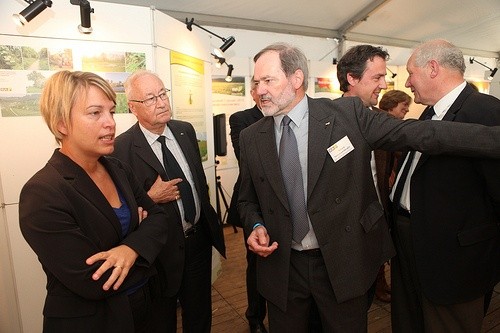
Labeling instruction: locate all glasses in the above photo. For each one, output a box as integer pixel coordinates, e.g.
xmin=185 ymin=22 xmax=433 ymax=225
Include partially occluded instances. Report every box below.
xmin=131 ymin=88 xmax=171 ymax=107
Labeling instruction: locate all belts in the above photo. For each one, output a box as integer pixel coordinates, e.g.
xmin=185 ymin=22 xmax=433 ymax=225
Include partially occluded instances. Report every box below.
xmin=184 ymin=221 xmax=203 ymax=239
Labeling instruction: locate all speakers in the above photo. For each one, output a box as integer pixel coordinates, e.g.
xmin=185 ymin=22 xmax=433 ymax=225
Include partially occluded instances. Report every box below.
xmin=213 ymin=114 xmax=228 ymax=156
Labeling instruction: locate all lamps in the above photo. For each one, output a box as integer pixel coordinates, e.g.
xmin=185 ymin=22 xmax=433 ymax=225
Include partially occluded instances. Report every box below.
xmin=70 ymin=0 xmax=94 ymax=34
xmin=12 ymin=0 xmax=52 ymax=25
xmin=470 ymin=57 xmax=498 ymax=80
xmin=211 ymin=53 xmax=234 ymax=82
xmin=185 ymin=17 xmax=236 ymax=53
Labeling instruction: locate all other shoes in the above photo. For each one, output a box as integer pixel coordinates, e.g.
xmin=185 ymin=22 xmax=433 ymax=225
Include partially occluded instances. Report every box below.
xmin=249 ymin=322 xmax=267 ymax=333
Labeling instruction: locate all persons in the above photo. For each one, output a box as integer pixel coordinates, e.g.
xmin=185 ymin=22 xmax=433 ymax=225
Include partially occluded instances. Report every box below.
xmin=105 ymin=71 xmax=226 ymax=333
xmin=389 ymin=38 xmax=500 ymax=333
xmin=337 ymin=45 xmax=388 ymax=309
xmin=228 ymin=75 xmax=267 ymax=333
xmin=19 ymin=71 xmax=171 ymax=333
xmin=238 ymin=42 xmax=500 ymax=333
xmin=375 ymin=90 xmax=412 ymax=303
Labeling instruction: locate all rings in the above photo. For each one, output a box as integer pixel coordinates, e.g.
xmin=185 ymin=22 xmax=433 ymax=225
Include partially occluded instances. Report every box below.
xmin=115 ymin=266 xmax=123 ymax=269
xmin=176 ymin=196 xmax=177 ymax=199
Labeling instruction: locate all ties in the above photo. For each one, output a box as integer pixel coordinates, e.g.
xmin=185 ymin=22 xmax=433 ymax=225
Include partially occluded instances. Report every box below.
xmin=157 ymin=135 xmax=196 ymax=225
xmin=391 ymin=106 xmax=435 ymax=231
xmin=279 ymin=116 xmax=310 ymax=246
